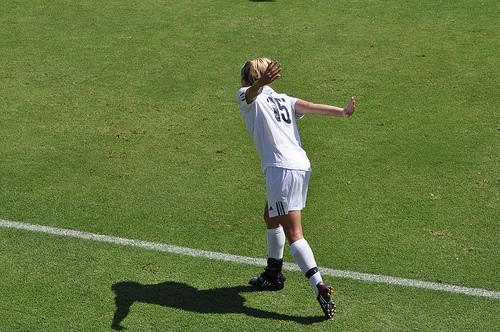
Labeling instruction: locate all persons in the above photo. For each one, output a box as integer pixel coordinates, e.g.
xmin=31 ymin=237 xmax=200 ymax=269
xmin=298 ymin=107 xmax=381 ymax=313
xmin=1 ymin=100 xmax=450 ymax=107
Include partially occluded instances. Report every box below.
xmin=236 ymin=58 xmax=357 ymax=319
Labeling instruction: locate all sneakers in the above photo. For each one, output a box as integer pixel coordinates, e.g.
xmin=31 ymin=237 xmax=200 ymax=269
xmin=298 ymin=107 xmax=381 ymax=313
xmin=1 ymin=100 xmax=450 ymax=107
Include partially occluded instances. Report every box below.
xmin=247 ymin=272 xmax=285 ymax=289
xmin=315 ymin=281 xmax=337 ymax=319
